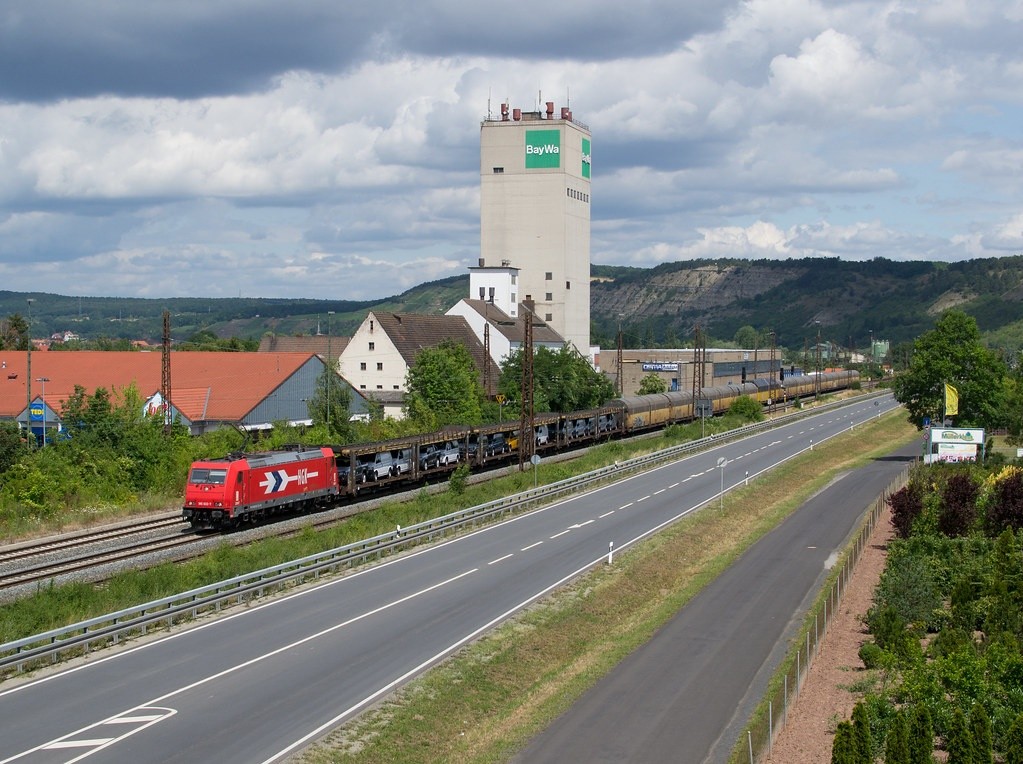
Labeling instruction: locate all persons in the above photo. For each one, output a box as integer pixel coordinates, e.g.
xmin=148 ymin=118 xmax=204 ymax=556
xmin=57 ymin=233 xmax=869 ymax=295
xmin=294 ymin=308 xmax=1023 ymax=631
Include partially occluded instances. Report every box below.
xmin=145 ymin=402 xmax=156 ymax=418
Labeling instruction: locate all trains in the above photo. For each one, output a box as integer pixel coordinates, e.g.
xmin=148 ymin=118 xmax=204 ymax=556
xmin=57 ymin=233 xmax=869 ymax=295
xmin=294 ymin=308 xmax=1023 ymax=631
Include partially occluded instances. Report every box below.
xmin=183 ymin=367 xmax=864 ymax=534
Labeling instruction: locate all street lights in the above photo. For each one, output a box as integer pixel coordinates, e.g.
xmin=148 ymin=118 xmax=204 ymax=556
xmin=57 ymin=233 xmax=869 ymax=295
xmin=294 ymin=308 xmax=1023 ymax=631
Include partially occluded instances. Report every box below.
xmin=618 ymin=314 xmax=625 ymax=350
xmin=28 ymin=299 xmax=42 ymax=448
xmin=815 ymin=320 xmax=821 ymax=397
xmin=869 ymin=330 xmax=873 ymax=390
xmin=327 ymin=310 xmax=336 ymax=438
xmin=35 ymin=378 xmax=50 ymax=449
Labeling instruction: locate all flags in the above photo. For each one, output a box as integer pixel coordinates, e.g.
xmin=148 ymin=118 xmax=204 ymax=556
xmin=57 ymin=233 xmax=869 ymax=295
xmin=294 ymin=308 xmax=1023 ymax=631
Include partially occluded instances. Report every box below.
xmin=945 ymin=384 xmax=959 ymax=416
